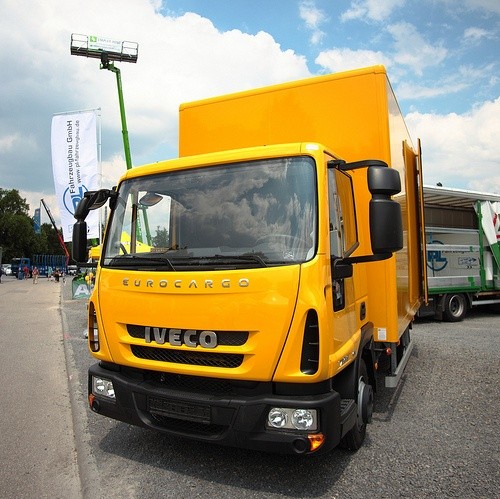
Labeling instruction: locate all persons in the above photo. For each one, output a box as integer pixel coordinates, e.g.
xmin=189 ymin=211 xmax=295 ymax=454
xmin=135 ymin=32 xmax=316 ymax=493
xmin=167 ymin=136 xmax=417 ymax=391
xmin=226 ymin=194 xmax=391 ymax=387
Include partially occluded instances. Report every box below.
xmin=47 ymin=264 xmax=65 ymax=285
xmin=0 ymin=268 xmax=2 ymax=282
xmin=23 ymin=266 xmax=29 ymax=280
xmin=32 ymin=267 xmax=39 ymax=284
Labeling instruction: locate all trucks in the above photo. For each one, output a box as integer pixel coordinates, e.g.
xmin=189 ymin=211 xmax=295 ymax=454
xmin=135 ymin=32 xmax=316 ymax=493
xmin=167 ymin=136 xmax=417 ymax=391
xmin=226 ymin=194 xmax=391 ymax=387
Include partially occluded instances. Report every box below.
xmin=0 ymin=255 xmax=67 ymax=278
xmin=68 ymin=63 xmax=500 ymax=457
